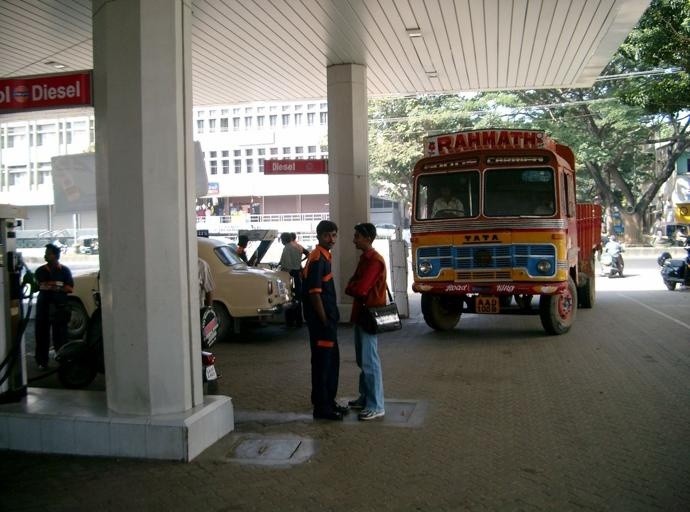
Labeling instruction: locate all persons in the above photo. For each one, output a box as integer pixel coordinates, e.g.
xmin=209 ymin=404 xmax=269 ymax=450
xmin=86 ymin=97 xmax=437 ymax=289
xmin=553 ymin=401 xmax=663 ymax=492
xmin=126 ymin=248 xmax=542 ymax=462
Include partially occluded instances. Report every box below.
xmin=34 ymin=243 xmax=75 ymax=370
xmin=604 ymin=234 xmax=625 ymax=276
xmin=235 ymin=237 xmax=248 ymax=262
xmin=431 ymin=186 xmax=465 ymax=218
xmin=302 ymin=220 xmax=349 ymax=421
xmin=198 ymin=256 xmax=215 ymax=309
xmin=344 ymin=223 xmax=387 ymax=421
xmin=280 ymin=233 xmax=301 ymax=282
xmin=291 ymin=232 xmax=310 ymax=261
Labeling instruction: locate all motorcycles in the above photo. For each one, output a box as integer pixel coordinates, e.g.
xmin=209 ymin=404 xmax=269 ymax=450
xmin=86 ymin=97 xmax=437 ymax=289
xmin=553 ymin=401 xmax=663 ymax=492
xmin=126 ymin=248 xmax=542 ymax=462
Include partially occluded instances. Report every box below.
xmin=53 ymin=290 xmax=219 ymax=398
xmin=601 ymin=242 xmax=626 ymax=277
xmin=657 ymin=247 xmax=690 ymax=291
xmin=668 ymin=235 xmax=690 ymax=246
xmin=58 ymin=237 xmax=98 ymax=255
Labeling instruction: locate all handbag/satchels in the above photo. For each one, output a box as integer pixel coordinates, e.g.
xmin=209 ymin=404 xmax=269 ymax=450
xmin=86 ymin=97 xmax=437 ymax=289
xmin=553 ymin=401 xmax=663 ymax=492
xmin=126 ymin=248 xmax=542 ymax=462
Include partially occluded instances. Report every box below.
xmin=363 ymin=302 xmax=403 ymax=335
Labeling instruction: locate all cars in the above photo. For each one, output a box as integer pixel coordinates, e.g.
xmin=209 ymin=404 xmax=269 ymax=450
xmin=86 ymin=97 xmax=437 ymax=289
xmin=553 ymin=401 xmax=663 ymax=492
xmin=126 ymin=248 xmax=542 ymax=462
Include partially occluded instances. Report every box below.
xmin=68 ymin=235 xmax=294 ymax=343
xmin=376 ymin=223 xmax=398 ymax=239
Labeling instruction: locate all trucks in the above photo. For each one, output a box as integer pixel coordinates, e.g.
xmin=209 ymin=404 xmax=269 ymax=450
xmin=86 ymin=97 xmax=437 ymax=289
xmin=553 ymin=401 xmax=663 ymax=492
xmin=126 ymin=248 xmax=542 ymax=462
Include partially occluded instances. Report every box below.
xmin=410 ymin=128 xmax=601 ymax=334
